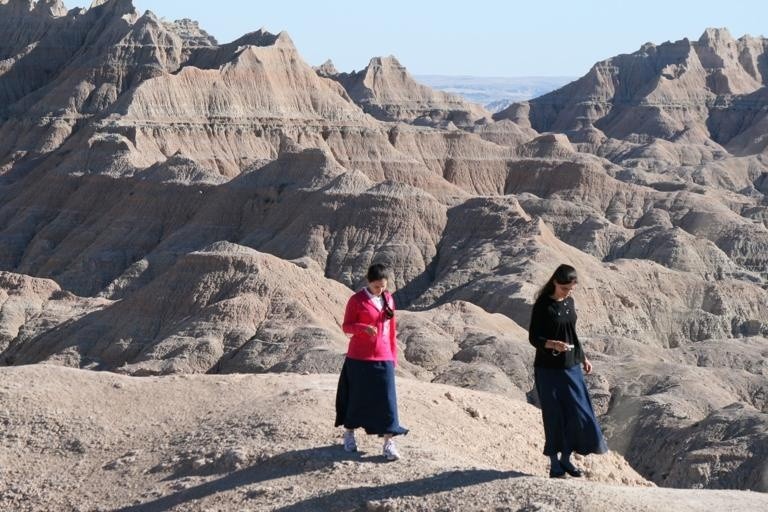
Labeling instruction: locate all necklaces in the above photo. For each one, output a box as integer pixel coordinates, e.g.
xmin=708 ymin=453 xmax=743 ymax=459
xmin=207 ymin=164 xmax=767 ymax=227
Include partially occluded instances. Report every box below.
xmin=553 ymin=298 xmax=563 ymax=316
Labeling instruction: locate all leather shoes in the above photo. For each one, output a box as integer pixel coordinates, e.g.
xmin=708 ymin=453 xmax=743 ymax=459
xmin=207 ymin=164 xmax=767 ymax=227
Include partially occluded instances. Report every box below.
xmin=558 ymin=461 xmax=584 ymax=477
xmin=550 ymin=468 xmax=566 ymax=478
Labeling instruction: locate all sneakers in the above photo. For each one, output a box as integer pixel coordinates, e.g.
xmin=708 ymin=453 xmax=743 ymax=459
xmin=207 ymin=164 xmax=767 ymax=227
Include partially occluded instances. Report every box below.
xmin=342 ymin=432 xmax=357 ymax=452
xmin=383 ymin=441 xmax=400 ymax=460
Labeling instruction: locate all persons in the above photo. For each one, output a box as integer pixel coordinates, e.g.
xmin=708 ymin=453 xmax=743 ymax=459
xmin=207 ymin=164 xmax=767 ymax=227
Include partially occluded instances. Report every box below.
xmin=335 ymin=263 xmax=408 ymax=461
xmin=528 ymin=265 xmax=606 ymax=477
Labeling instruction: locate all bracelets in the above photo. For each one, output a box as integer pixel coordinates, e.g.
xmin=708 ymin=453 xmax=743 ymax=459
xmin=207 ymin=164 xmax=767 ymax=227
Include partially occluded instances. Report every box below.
xmin=553 ymin=341 xmax=556 ymax=351
